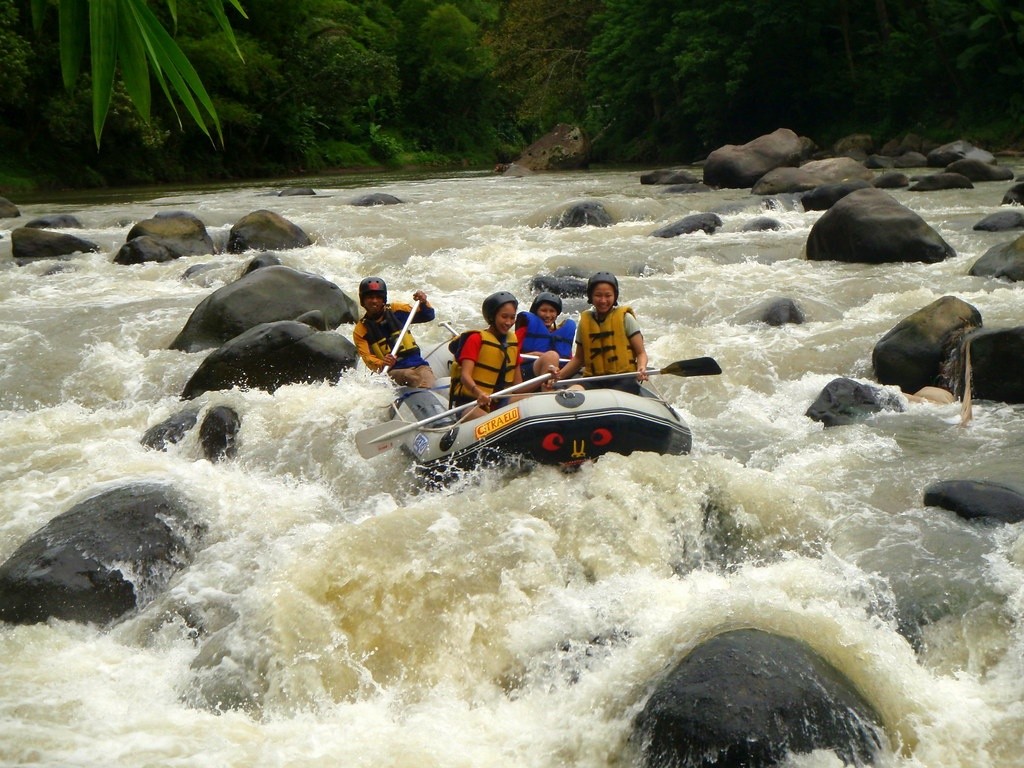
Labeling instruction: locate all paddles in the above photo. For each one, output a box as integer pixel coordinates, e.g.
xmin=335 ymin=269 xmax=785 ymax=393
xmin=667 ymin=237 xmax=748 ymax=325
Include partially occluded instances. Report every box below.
xmin=545 ymin=355 xmax=723 ymax=384
xmin=375 ymin=293 xmax=422 ymax=379
xmin=354 ymin=364 xmax=556 ymax=461
xmin=438 ymin=318 xmax=459 ymax=337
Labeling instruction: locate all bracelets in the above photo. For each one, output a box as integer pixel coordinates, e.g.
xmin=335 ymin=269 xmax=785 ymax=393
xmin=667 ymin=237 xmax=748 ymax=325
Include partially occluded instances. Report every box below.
xmin=470 ymin=384 xmax=479 ymax=394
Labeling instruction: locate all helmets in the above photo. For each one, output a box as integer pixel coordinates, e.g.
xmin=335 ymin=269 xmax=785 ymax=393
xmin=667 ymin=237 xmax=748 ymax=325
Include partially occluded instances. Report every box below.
xmin=587 ymin=271 xmax=619 ymax=306
xmin=482 ymin=292 xmax=518 ymax=326
xmin=360 ymin=277 xmax=387 ymax=306
xmin=530 ymin=292 xmax=562 ymax=316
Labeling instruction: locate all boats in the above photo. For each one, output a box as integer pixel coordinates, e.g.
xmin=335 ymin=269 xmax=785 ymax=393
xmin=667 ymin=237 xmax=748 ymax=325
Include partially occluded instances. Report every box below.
xmin=385 ymin=375 xmax=692 ymax=489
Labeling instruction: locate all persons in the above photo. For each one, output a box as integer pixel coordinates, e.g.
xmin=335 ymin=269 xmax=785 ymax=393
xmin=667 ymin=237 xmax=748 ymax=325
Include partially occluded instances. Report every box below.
xmin=443 ymin=292 xmax=562 ymax=424
xmin=349 ymin=275 xmax=450 ymax=426
xmin=508 ymin=289 xmax=576 ymax=393
xmin=554 ymin=271 xmax=649 ymax=394
